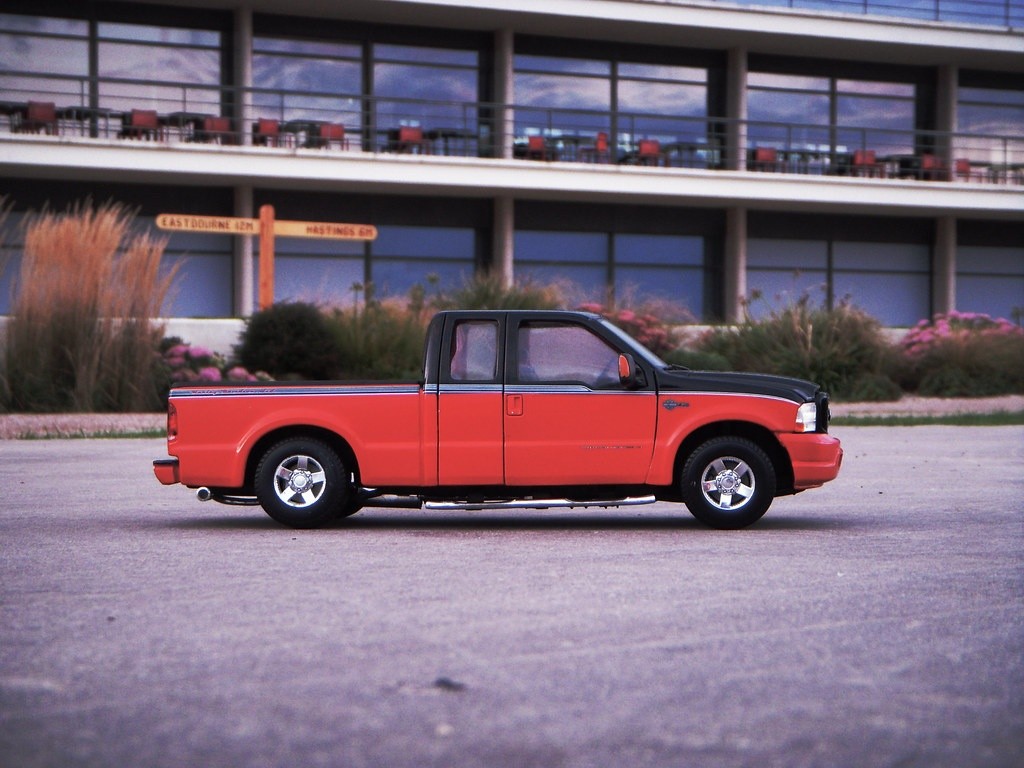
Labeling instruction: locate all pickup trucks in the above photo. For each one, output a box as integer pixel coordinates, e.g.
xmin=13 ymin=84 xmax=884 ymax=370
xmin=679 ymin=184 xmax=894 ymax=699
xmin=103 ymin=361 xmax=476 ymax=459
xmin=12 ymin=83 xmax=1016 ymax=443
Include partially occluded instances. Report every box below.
xmin=152 ymin=308 xmax=843 ymax=529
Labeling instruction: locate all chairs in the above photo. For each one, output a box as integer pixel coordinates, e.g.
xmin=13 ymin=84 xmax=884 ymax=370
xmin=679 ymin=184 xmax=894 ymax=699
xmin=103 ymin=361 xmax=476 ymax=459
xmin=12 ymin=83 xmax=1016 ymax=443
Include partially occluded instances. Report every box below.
xmin=519 ymin=349 xmax=539 ymax=382
xmin=0 ymin=100 xmax=1023 ymax=187
